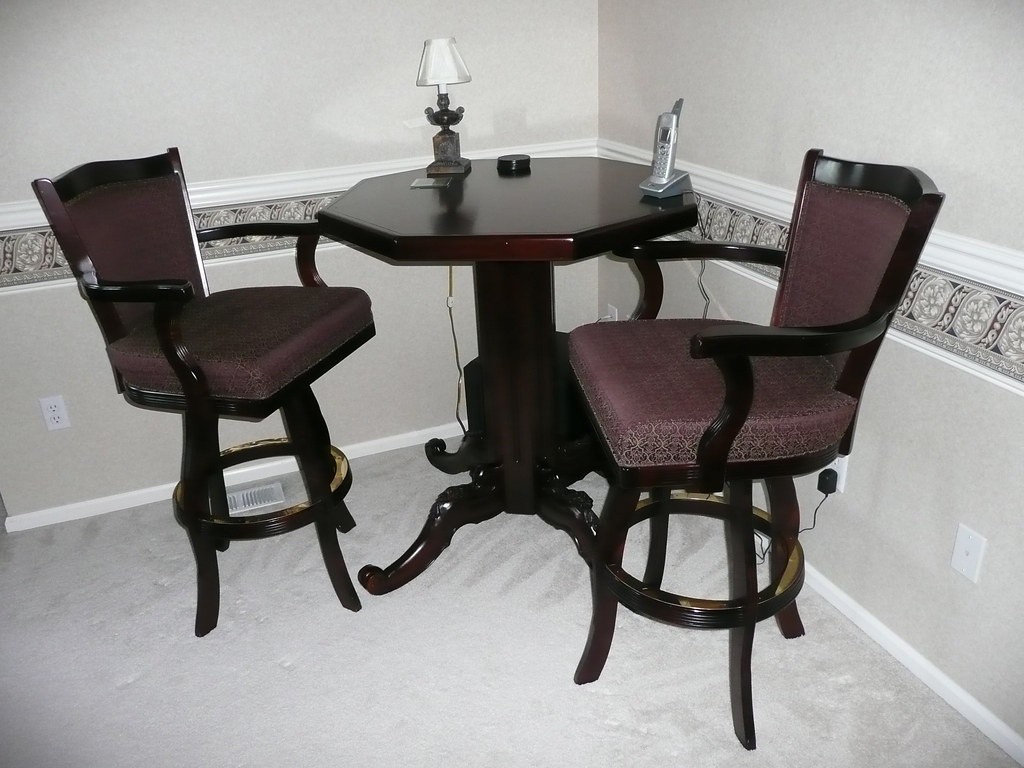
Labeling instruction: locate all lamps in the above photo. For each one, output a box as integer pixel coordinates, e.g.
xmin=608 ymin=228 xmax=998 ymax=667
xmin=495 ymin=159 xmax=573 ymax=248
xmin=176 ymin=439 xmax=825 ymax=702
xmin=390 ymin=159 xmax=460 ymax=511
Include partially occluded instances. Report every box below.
xmin=415 ymin=36 xmax=472 ymax=177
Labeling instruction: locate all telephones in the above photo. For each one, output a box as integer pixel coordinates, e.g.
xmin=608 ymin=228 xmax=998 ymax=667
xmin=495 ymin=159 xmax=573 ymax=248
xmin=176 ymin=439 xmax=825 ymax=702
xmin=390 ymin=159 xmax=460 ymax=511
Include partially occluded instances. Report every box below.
xmin=637 ymin=97 xmax=692 ymax=199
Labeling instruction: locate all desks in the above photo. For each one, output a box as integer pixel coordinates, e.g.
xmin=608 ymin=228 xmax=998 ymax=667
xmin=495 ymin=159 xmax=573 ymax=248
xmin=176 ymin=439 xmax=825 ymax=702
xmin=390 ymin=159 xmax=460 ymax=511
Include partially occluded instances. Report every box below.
xmin=315 ymin=157 xmax=699 ymax=596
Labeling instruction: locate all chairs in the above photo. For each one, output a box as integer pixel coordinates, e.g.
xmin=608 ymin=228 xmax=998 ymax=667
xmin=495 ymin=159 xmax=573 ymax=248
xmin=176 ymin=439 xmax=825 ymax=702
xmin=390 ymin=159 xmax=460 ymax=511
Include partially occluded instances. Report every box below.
xmin=573 ymin=148 xmax=945 ymax=752
xmin=30 ymin=145 xmax=377 ymax=638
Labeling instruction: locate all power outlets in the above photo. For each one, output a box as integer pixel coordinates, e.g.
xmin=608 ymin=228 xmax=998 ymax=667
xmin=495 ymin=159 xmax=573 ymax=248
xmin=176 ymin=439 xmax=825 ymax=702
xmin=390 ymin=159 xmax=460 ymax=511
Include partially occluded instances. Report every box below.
xmin=950 ymin=522 xmax=986 ymax=582
xmin=819 ymin=454 xmax=848 ymax=494
xmin=604 ymin=304 xmax=617 ymax=322
xmin=39 ymin=394 xmax=69 ymax=431
